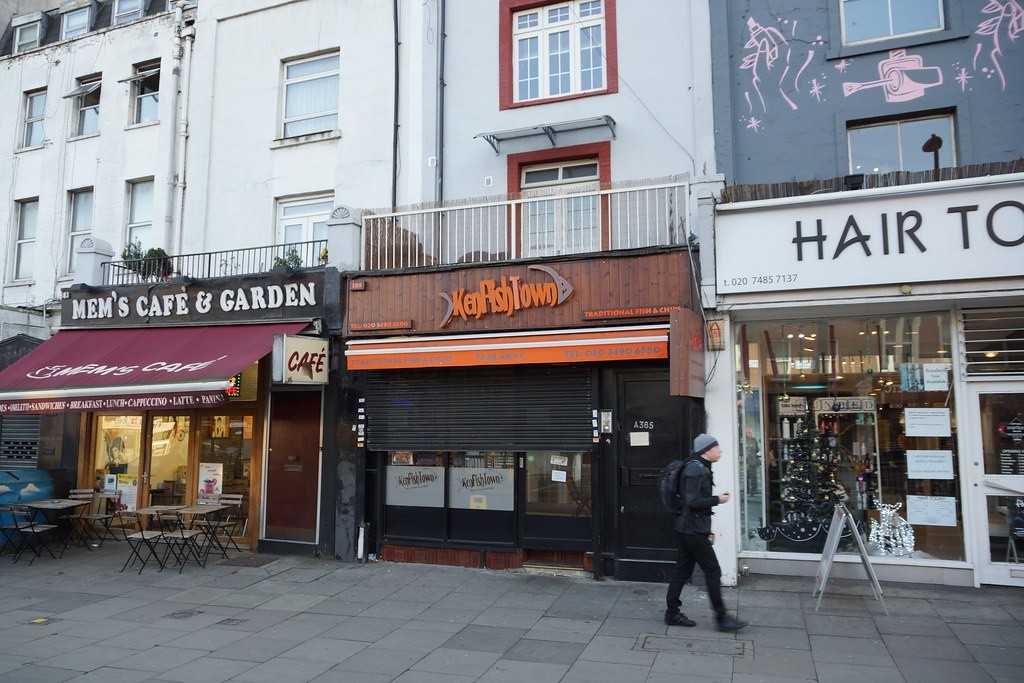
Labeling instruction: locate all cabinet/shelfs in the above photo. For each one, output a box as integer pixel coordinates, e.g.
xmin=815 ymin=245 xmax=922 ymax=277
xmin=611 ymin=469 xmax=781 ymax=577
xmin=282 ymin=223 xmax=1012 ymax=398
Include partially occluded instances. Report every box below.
xmin=774 ymin=396 xmax=884 ymax=543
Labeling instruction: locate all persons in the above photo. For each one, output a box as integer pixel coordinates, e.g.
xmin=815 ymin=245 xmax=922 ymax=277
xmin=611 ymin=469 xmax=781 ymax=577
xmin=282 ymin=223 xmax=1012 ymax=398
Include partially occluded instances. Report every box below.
xmin=166 ymin=416 xmax=178 ymax=439
xmin=657 ymin=433 xmax=750 ymax=632
xmin=394 ymin=451 xmax=411 ymax=463
xmin=212 ymin=416 xmax=225 ymax=437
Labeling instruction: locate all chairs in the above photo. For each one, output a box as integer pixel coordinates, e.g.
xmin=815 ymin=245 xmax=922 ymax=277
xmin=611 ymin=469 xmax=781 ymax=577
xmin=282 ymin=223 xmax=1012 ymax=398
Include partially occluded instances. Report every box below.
xmin=0 ymin=503 xmax=60 ymax=564
xmin=120 ymin=491 xmax=246 ymax=577
xmin=63 ymin=487 xmax=125 ymax=552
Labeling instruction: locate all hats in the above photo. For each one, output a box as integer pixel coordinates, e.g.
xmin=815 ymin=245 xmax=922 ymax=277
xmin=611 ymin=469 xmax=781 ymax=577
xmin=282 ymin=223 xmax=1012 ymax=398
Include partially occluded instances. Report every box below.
xmin=693 ymin=433 xmax=718 ymax=455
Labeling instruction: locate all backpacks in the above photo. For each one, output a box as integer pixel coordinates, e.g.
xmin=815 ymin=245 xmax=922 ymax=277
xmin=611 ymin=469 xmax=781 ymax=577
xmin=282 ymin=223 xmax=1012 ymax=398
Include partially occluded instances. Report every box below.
xmin=659 ymin=460 xmax=708 ymax=508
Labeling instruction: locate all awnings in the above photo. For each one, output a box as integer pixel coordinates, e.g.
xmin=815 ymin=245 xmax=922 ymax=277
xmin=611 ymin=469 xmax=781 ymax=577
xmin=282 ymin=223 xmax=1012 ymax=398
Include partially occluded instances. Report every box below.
xmin=0 ymin=320 xmax=313 ymax=415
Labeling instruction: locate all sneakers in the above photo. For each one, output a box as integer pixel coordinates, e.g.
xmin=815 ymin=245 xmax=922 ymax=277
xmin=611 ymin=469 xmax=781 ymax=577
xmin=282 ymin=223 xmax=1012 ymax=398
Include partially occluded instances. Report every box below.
xmin=665 ymin=612 xmax=696 ymax=627
xmin=718 ymin=616 xmax=747 ymax=631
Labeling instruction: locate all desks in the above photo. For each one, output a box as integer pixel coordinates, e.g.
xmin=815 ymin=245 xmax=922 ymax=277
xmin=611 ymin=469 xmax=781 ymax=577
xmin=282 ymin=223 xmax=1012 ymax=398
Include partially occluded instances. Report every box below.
xmin=134 ymin=505 xmax=233 ymax=561
xmin=10 ymin=498 xmax=91 ymax=558
